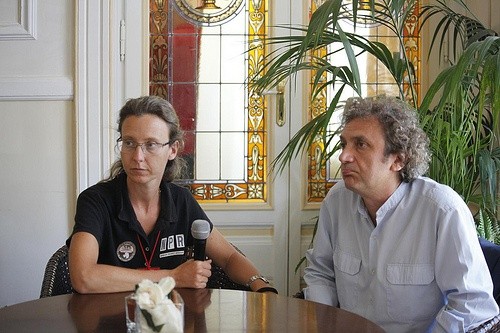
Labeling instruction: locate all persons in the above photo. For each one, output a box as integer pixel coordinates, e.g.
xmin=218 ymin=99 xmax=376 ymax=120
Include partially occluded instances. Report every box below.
xmin=68 ymin=288 xmax=212 ymax=333
xmin=302 ymin=95 xmax=500 ymax=333
xmin=65 ymin=96 xmax=279 ymax=295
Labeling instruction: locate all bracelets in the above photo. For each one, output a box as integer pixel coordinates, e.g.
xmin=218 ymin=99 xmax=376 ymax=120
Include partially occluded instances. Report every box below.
xmin=257 ymin=287 xmax=278 ymax=294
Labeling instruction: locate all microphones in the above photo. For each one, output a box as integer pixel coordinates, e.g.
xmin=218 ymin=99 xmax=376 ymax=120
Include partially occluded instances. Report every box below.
xmin=190 ymin=219 xmax=210 ymax=260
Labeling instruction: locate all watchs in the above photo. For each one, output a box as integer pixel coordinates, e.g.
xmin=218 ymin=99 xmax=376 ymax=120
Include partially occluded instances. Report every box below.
xmin=245 ymin=274 xmax=269 ymax=290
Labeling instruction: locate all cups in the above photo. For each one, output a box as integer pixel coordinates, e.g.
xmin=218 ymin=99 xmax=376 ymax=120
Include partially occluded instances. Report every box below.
xmin=123 ymin=289 xmax=185 ymax=333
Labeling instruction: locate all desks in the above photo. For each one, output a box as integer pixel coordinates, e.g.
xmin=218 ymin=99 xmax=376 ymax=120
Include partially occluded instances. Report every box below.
xmin=0 ymin=287 xmax=386 ymax=333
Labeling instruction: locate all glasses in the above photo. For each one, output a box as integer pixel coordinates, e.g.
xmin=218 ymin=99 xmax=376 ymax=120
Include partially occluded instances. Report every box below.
xmin=116 ymin=136 xmax=172 ymax=157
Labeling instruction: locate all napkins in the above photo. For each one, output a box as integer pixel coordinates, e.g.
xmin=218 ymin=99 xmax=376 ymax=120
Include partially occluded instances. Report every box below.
xmin=134 ymin=276 xmax=182 ymax=333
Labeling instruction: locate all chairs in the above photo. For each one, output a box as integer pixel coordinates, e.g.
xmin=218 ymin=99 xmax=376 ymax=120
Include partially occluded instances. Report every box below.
xmin=39 ymin=245 xmax=251 ymax=298
